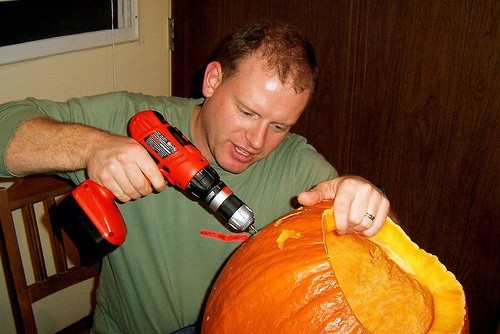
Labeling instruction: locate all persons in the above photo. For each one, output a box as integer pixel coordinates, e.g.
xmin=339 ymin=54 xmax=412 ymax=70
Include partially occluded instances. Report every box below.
xmin=0 ymin=23 xmax=392 ymax=333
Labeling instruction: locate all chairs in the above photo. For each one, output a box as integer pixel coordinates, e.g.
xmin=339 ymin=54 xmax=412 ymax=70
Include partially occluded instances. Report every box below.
xmin=0 ymin=172 xmax=103 ymax=334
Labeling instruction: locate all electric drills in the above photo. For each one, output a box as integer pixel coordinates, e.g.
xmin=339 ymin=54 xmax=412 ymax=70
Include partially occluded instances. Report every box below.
xmin=47 ymin=109 xmax=258 ymax=272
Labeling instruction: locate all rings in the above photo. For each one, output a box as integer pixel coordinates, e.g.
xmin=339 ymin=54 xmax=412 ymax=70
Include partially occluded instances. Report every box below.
xmin=364 ymin=212 xmax=376 ymax=221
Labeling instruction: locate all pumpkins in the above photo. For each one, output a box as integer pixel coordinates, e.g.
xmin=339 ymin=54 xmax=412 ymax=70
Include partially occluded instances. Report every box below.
xmin=200 ymin=199 xmax=471 ymax=334
